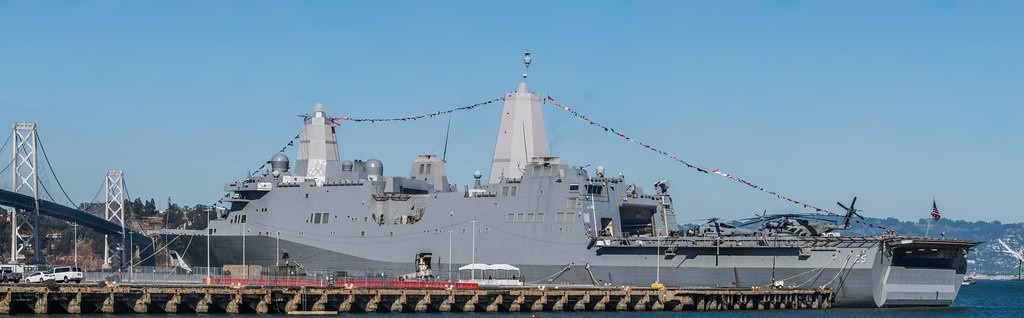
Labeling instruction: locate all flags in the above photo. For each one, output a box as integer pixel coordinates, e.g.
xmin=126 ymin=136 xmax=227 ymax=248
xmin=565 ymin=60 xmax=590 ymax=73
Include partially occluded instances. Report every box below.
xmin=931 ymin=200 xmax=941 ymax=222
xmin=331 ymin=120 xmax=341 ymax=128
xmin=548 ymin=95 xmax=554 ymax=103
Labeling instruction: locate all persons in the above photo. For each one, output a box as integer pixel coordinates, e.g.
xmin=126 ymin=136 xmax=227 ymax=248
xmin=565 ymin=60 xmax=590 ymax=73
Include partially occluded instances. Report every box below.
xmin=686 ymin=229 xmax=698 ymax=237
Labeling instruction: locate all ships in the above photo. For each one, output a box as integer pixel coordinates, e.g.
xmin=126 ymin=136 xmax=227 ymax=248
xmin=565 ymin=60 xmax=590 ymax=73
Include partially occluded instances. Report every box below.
xmin=147 ymin=52 xmax=989 ymax=309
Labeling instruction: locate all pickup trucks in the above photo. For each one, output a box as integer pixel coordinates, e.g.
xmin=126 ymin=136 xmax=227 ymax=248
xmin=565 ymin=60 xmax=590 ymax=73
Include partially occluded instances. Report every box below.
xmin=0 ymin=269 xmax=22 ymax=283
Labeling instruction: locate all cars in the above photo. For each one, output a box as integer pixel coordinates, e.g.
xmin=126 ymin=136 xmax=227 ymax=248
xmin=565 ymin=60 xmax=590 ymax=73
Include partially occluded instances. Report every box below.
xmin=24 ymin=271 xmax=55 ymax=284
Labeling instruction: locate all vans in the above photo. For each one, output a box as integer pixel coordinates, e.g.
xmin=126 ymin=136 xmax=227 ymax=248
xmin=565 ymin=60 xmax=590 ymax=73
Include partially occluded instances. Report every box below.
xmin=49 ymin=265 xmax=83 ymax=284
